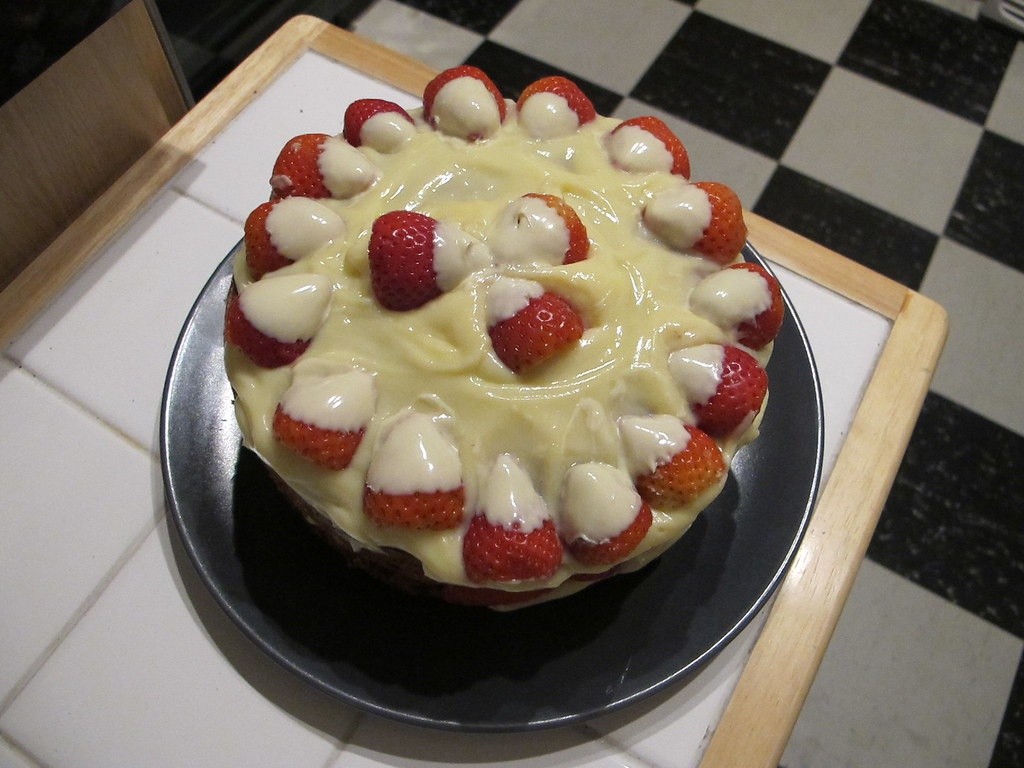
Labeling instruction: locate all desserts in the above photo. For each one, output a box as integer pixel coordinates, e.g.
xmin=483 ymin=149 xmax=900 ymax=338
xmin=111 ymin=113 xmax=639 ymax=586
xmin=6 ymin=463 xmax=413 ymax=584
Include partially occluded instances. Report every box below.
xmin=224 ymin=63 xmax=782 ymax=613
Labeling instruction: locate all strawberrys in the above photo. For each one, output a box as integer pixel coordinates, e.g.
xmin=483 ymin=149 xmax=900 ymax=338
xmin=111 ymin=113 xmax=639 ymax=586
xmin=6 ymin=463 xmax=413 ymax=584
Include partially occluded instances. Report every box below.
xmin=225 ymin=68 xmax=784 ymax=581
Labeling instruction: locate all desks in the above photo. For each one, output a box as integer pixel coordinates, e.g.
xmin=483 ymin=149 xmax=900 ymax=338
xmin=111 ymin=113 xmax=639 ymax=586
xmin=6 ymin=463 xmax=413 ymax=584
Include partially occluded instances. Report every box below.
xmin=229 ymin=0 xmax=1024 ymax=767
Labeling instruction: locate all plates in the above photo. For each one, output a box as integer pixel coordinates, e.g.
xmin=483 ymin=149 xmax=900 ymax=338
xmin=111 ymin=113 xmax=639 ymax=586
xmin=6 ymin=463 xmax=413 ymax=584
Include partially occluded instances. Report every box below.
xmin=164 ymin=230 xmax=824 ymax=735
